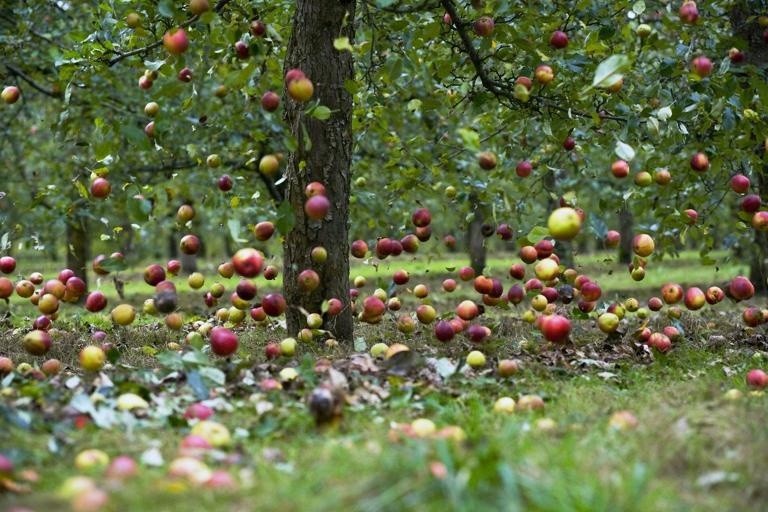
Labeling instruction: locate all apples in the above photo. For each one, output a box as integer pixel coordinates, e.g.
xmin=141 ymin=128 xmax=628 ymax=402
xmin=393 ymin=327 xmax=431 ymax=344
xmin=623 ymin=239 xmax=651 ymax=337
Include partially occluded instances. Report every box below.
xmin=0 ymin=1 xmax=768 ymax=512
